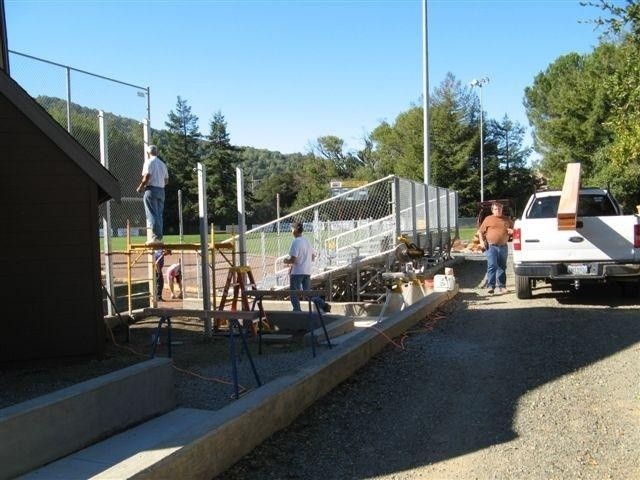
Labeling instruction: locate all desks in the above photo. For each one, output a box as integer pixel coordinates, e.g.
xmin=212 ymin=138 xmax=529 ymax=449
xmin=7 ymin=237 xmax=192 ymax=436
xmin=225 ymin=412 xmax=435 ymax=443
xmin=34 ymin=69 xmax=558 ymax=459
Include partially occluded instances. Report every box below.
xmin=471 ymin=76 xmax=491 ymax=200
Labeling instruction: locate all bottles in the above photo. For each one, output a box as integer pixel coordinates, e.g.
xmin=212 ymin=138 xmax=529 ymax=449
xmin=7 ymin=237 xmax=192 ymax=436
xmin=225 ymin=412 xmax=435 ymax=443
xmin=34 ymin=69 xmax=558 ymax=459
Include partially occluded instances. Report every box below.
xmin=289 ymin=223 xmax=303 ymax=231
xmin=166 ymin=249 xmax=172 ymax=255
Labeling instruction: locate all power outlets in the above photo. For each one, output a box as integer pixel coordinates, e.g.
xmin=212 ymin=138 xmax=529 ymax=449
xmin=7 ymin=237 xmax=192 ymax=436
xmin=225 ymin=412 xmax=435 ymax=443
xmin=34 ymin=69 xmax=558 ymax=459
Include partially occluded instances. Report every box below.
xmin=513 ymin=188 xmax=640 ymax=300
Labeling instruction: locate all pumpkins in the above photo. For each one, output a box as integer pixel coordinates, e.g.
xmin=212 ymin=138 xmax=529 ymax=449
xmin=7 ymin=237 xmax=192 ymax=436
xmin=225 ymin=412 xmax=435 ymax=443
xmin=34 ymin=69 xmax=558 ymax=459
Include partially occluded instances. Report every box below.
xmin=214 ymin=267 xmax=273 ymax=342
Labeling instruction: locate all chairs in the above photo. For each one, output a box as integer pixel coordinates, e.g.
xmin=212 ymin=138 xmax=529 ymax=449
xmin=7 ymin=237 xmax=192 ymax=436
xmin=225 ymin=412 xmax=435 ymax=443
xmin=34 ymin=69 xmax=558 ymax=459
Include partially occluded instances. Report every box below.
xmin=156 ymin=293 xmax=184 ymax=302
xmin=322 ymin=303 xmax=331 ymax=312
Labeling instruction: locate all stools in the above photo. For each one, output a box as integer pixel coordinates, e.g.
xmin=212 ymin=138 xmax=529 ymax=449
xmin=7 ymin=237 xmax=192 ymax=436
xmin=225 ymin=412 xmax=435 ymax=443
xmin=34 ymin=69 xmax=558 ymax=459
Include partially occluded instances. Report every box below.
xmin=487 ymin=243 xmax=507 ymax=247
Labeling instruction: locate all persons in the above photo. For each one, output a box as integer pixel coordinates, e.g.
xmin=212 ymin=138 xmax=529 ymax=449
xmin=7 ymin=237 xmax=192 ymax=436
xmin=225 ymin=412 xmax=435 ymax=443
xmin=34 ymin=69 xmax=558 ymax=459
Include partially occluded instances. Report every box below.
xmin=154 ymin=249 xmax=173 ymax=302
xmin=136 ymin=144 xmax=169 ymax=246
xmin=283 ymin=222 xmax=331 ymax=315
xmin=478 ymin=201 xmax=514 ymax=294
xmin=167 ymin=258 xmax=183 ymax=298
